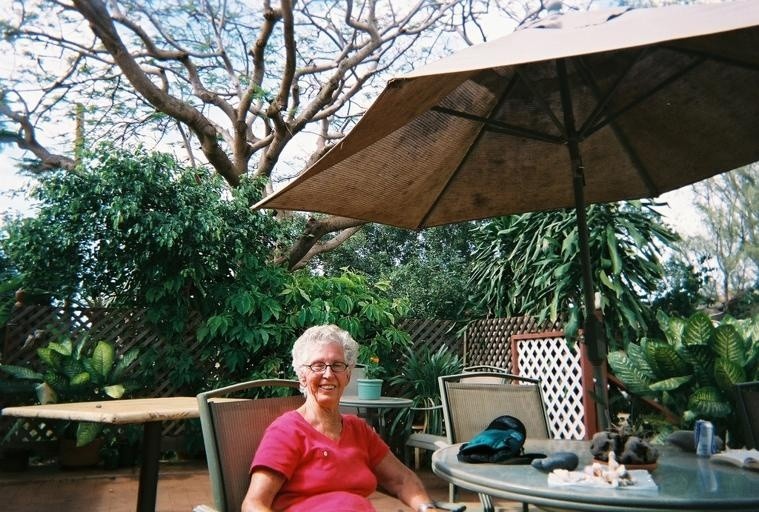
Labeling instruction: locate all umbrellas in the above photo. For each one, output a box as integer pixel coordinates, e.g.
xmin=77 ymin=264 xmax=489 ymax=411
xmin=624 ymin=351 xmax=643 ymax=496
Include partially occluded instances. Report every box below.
xmin=248 ymin=0 xmax=759 ymax=431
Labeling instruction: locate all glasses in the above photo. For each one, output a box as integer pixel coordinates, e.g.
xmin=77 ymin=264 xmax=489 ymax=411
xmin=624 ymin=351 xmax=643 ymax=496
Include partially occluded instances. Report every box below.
xmin=302 ymin=360 xmax=349 ymax=372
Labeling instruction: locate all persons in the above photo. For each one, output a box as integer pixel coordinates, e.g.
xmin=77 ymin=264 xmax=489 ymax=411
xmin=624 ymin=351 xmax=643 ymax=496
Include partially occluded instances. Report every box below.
xmin=242 ymin=325 xmax=447 ymax=512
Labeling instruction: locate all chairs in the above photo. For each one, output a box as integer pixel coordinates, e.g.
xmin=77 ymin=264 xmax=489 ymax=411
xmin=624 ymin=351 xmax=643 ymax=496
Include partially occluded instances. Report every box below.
xmin=405 ymin=366 xmax=554 ymax=511
xmin=197 ymin=377 xmax=466 ymax=511
xmin=334 ymin=366 xmax=368 ymax=419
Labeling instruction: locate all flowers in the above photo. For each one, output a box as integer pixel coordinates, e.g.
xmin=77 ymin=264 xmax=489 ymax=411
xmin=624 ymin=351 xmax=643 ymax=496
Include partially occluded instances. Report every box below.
xmin=365 ymin=356 xmax=386 ymax=379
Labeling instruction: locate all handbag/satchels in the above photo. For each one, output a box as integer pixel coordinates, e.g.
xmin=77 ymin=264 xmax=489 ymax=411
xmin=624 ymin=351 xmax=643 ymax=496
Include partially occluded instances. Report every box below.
xmin=457 ymin=415 xmax=546 ymax=465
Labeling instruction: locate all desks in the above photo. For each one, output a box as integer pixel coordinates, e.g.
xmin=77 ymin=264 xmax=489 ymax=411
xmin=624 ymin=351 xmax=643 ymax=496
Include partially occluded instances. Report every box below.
xmin=337 ymin=393 xmax=414 ymax=437
xmin=2 ymin=395 xmax=254 ymax=512
xmin=429 ymin=439 xmax=759 ymax=510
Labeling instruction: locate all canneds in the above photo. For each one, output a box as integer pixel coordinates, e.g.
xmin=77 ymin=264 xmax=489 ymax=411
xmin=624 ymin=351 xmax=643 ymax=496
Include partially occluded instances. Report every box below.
xmin=694 ymin=420 xmax=716 ymax=457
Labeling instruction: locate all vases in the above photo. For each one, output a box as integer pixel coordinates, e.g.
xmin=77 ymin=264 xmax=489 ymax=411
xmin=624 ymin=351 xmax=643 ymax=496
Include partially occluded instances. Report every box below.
xmin=355 ymin=377 xmax=385 ymax=400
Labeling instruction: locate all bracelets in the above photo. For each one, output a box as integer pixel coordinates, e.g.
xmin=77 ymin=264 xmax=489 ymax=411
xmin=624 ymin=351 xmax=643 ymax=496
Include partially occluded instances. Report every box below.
xmin=418 ymin=503 xmax=436 ymax=512
